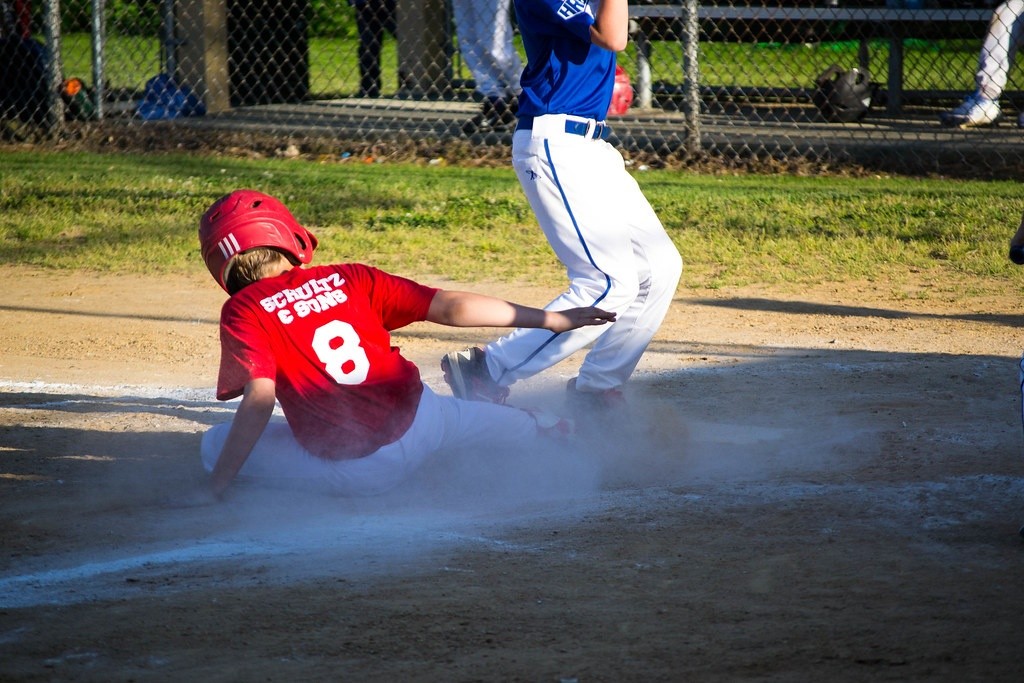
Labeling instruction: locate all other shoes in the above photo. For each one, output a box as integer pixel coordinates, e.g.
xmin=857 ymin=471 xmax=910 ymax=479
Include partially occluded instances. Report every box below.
xmin=472 ymin=98 xmax=519 ymax=130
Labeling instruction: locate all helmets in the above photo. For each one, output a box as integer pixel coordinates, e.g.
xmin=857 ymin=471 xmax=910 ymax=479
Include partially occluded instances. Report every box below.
xmin=198 ymin=188 xmax=318 ymax=296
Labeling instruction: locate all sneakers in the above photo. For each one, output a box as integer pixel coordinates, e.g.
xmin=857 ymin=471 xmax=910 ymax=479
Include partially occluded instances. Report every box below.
xmin=566 ymin=378 xmax=629 ymax=426
xmin=939 ymin=96 xmax=1003 ymax=127
xmin=440 ymin=345 xmax=510 ymax=405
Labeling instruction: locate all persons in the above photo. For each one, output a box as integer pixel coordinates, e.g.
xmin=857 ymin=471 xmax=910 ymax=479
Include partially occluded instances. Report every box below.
xmin=171 ymin=189 xmax=633 ymax=510
xmin=345 ymin=0 xmax=396 ymax=98
xmin=440 ymin=0 xmax=684 ymax=416
xmin=942 ymin=0 xmax=1024 ymax=267
xmin=452 ymin=0 xmax=524 ymax=135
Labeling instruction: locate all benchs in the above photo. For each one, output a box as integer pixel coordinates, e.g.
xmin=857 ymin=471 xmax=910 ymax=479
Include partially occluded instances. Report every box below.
xmin=628 ymin=4 xmax=995 ymax=115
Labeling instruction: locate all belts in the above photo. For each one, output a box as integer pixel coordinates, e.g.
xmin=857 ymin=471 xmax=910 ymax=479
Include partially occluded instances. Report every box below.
xmin=516 ymin=115 xmax=611 ymax=140
